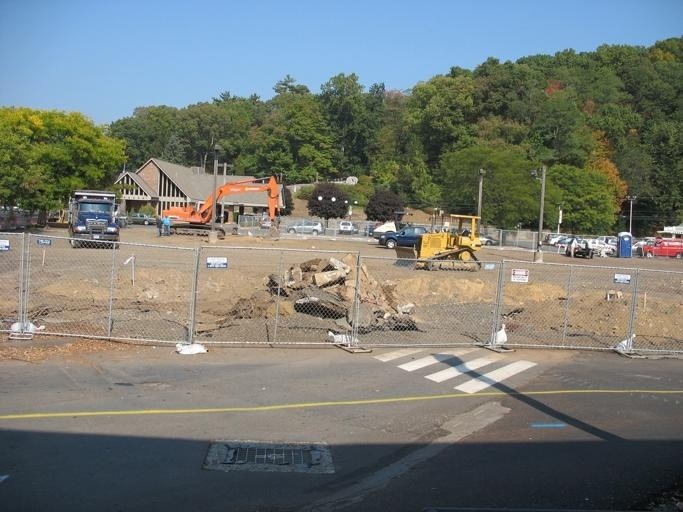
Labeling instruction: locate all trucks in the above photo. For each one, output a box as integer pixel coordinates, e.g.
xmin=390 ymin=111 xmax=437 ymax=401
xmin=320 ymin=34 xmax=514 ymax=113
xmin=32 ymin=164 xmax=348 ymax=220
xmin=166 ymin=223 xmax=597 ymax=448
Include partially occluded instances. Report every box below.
xmin=66 ymin=187 xmax=119 ymax=249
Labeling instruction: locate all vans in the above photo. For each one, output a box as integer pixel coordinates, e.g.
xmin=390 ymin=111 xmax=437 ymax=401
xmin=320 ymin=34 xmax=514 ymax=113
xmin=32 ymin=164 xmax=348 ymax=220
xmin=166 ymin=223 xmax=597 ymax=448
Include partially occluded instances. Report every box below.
xmin=639 ymin=238 xmax=682 ymax=260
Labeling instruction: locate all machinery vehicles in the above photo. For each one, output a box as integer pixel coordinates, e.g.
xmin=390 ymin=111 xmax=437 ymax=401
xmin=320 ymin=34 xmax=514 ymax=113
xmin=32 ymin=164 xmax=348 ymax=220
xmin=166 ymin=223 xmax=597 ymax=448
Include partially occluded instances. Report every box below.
xmin=393 ymin=211 xmax=483 ymax=273
xmin=162 ymin=173 xmax=283 ymax=236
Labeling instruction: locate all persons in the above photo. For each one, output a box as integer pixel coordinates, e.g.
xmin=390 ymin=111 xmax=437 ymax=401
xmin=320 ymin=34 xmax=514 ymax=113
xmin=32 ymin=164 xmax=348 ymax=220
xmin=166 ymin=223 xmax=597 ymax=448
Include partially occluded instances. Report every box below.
xmin=570 ymin=236 xmax=579 ymax=257
xmin=156 ymin=215 xmax=163 ymax=237
xmin=162 ymin=214 xmax=171 ymax=236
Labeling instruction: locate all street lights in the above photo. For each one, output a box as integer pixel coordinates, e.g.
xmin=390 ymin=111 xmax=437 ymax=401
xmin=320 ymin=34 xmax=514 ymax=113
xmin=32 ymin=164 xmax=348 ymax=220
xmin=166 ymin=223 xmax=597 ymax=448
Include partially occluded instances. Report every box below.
xmin=477 ymin=167 xmax=486 ymax=232
xmin=531 ymin=165 xmax=547 ymax=263
xmin=626 ymin=194 xmax=638 ymax=234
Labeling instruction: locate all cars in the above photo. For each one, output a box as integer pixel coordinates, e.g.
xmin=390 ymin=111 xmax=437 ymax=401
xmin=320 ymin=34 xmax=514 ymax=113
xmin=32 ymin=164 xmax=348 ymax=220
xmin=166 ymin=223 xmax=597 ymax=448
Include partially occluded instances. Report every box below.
xmin=546 ymin=231 xmax=621 ymax=258
xmin=259 ymin=211 xmax=360 ymax=238
xmin=378 ymin=226 xmax=428 ymax=249
xmin=479 ymin=233 xmax=499 ymax=246
xmin=132 ymin=211 xmax=156 ymax=226
